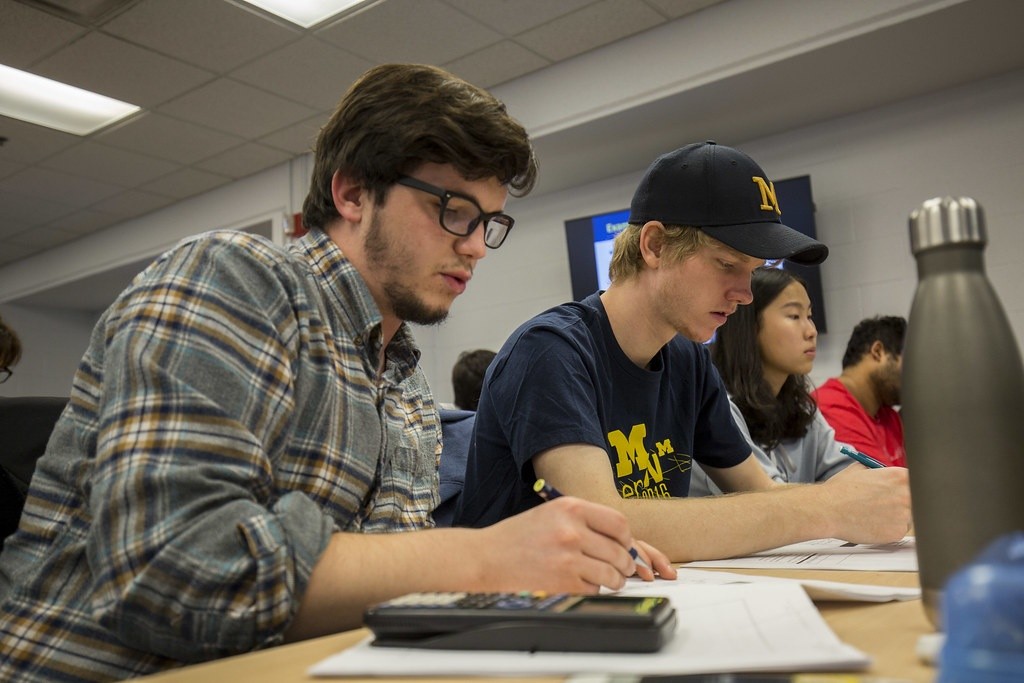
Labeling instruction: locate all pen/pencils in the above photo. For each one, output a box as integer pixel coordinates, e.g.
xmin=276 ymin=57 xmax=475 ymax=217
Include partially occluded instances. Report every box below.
xmin=531 ymin=478 xmax=655 ymax=574
xmin=840 ymin=446 xmax=888 ymax=468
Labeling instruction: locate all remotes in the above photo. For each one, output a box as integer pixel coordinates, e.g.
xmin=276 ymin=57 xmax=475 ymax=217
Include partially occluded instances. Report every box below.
xmin=365 ymin=588 xmax=677 ymax=654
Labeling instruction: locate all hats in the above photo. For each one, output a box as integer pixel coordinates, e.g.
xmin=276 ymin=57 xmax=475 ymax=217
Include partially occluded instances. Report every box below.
xmin=627 ymin=140 xmax=829 ymax=266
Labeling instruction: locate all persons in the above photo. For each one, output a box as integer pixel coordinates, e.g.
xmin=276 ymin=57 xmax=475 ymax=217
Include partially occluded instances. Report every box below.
xmin=0 ymin=65 xmax=678 ymax=682
xmin=452 ymin=140 xmax=916 ymax=563
xmin=687 ymin=265 xmax=860 ymax=496
xmin=432 ymin=350 xmax=498 ymax=528
xmin=811 ymin=315 xmax=909 ymax=467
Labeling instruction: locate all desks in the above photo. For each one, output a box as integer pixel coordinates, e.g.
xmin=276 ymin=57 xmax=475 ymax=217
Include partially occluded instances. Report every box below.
xmin=122 ymin=535 xmax=943 ymax=683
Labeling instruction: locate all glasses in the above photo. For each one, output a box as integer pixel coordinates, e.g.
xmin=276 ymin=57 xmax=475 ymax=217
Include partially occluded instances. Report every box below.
xmin=0 ymin=365 xmax=13 ymax=384
xmin=393 ymin=174 xmax=514 ymax=250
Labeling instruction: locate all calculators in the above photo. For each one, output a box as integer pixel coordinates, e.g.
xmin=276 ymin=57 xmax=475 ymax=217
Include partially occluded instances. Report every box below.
xmin=362 ymin=588 xmax=679 ymax=653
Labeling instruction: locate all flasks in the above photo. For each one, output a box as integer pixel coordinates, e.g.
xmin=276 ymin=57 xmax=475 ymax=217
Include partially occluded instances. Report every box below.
xmin=903 ymin=196 xmax=1023 ymax=626
